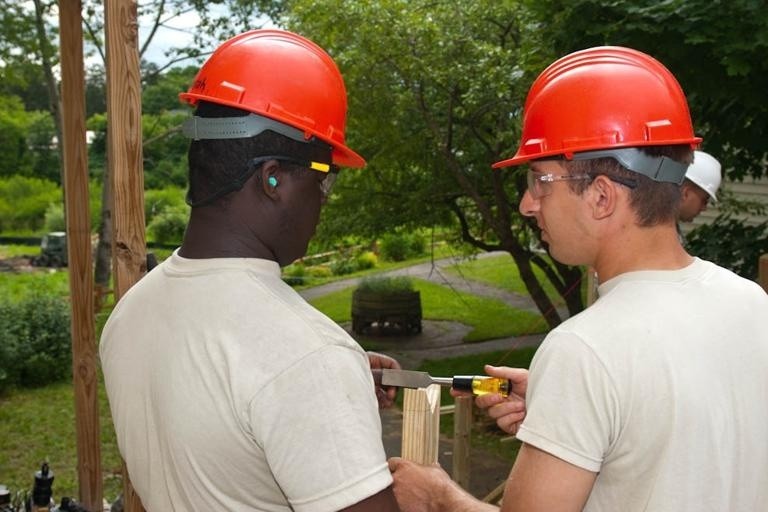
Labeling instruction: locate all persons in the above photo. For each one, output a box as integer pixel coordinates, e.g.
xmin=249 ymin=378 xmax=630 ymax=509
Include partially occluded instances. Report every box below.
xmin=384 ymin=43 xmax=767 ymax=512
xmin=678 ymin=151 xmax=722 ymax=224
xmin=94 ymin=29 xmax=399 ymax=512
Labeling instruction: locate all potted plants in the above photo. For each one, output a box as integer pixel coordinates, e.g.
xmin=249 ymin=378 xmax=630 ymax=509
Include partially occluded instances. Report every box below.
xmin=348 ymin=271 xmax=422 ymax=336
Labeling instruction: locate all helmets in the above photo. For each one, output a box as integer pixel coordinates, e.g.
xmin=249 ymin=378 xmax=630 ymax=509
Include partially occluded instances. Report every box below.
xmin=685 ymin=152 xmax=722 ymax=205
xmin=492 ymin=47 xmax=701 ymax=168
xmin=178 ymin=28 xmax=367 ymax=168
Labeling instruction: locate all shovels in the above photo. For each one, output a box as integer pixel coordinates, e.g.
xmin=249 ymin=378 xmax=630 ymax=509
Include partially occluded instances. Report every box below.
xmin=382 ymin=368 xmax=512 ymax=397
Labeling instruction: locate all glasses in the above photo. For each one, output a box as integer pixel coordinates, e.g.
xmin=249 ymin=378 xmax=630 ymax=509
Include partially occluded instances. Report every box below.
xmin=247 ymin=154 xmax=340 ymax=195
xmin=526 ymin=169 xmax=636 ymax=200
